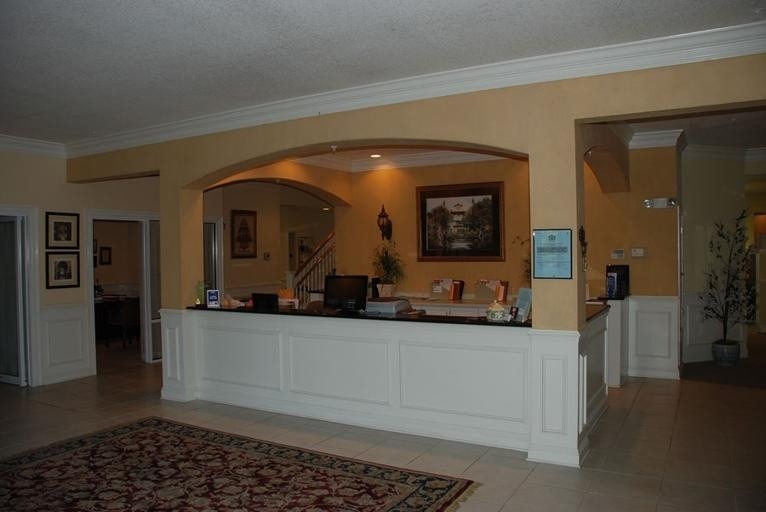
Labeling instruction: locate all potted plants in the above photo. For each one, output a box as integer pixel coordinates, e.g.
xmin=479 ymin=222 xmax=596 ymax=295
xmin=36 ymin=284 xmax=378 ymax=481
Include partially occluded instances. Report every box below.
xmin=696 ymin=209 xmax=759 ymax=369
xmin=368 ymin=240 xmax=410 ymax=298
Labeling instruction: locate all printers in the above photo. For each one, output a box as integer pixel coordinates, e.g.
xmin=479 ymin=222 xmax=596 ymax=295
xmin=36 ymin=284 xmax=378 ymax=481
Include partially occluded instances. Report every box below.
xmin=365 ymin=296 xmax=413 ymax=314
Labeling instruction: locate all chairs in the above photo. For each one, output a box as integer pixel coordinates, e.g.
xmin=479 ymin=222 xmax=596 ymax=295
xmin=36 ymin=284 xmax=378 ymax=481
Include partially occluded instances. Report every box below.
xmin=100 ymin=294 xmax=132 ymax=349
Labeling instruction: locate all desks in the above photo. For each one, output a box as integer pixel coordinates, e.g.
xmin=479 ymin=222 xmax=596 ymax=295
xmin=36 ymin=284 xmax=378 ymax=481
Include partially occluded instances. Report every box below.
xmin=93 ymin=292 xmax=140 ymax=346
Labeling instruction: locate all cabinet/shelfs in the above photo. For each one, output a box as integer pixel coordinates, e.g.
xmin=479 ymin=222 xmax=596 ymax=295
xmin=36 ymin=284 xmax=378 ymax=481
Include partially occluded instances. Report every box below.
xmin=479 ymin=300 xmax=516 ymax=318
xmin=308 ymin=289 xmax=323 ymax=303
xmin=399 ymin=293 xmax=427 ymax=312
xmin=422 ymin=299 xmax=478 ymax=318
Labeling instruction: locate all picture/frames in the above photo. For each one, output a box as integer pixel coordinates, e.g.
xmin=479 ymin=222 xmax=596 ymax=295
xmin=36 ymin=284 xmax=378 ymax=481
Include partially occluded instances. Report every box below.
xmin=44 ymin=252 xmax=80 ymax=290
xmin=415 ymin=181 xmax=506 ymax=263
xmin=531 ymin=228 xmax=573 ymax=280
xmin=44 ymin=211 xmax=80 ymax=251
xmin=230 ymin=209 xmax=256 ymax=259
xmin=92 ymin=239 xmax=111 ymax=269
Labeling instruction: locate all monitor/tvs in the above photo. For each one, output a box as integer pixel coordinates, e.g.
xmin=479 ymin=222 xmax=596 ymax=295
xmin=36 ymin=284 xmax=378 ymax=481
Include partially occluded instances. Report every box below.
xmin=252 ymin=292 xmax=278 ymax=308
xmin=323 ymin=275 xmax=368 ymax=313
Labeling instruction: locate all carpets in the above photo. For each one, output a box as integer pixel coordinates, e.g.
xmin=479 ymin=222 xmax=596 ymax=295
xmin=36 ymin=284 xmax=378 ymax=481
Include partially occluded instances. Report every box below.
xmin=0 ymin=417 xmax=481 ymax=512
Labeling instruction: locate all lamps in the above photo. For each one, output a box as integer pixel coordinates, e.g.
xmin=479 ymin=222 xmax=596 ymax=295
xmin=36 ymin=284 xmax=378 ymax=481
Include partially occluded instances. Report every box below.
xmin=376 ymin=204 xmax=392 ymax=242
